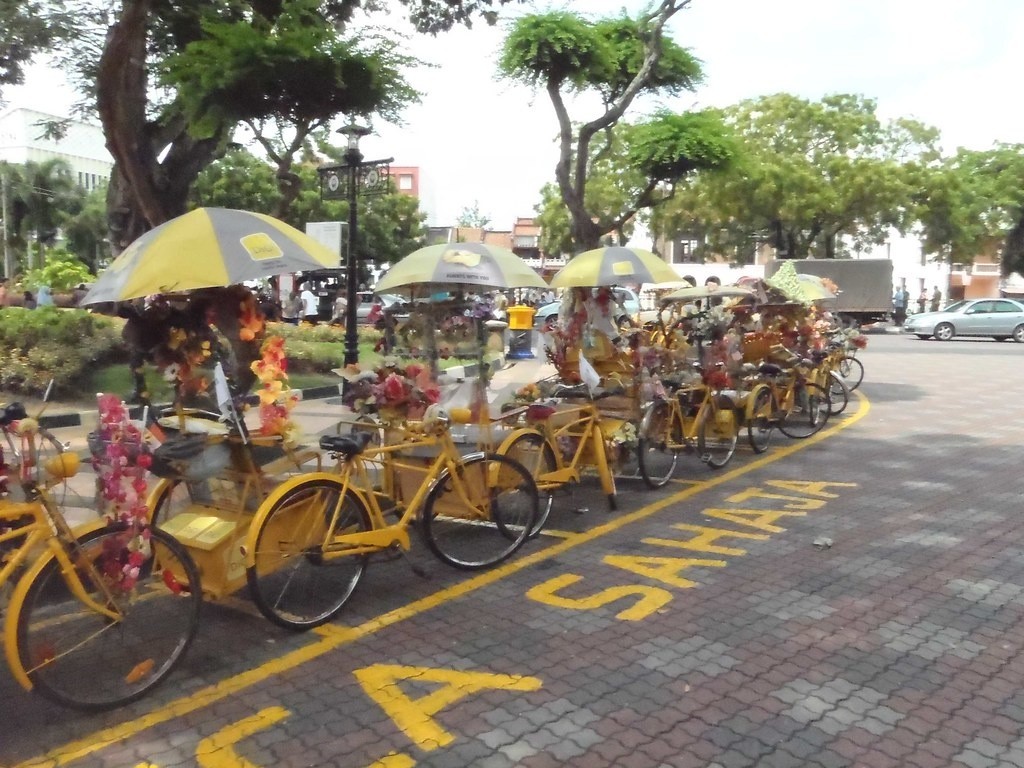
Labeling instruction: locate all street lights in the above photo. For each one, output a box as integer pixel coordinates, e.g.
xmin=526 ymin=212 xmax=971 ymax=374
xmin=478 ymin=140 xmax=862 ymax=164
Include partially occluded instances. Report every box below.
xmin=317 ymin=120 xmax=394 ymax=406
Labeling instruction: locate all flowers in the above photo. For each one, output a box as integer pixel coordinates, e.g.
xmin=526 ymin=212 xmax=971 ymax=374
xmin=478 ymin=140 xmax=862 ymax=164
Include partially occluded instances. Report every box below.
xmin=91 ymin=293 xmax=836 ymax=599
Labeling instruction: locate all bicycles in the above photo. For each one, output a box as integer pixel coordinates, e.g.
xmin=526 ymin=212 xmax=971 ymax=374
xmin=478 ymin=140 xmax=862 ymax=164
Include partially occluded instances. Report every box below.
xmin=240 ymin=411 xmax=539 ymax=631
xmin=745 ymin=353 xmax=831 ymax=453
xmin=0 ymin=410 xmax=204 ymax=714
xmin=823 ymin=344 xmax=865 ymax=396
xmin=637 ymin=360 xmax=740 ymax=490
xmin=808 ymin=348 xmax=849 ymax=428
xmin=0 ymin=402 xmax=232 ymax=572
xmin=488 ymin=372 xmax=628 ymax=543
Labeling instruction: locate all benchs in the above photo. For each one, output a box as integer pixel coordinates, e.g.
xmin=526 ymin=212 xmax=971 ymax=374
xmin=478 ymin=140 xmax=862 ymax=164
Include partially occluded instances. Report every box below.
xmin=160 ymin=409 xmax=324 ymax=475
xmin=710 ymin=390 xmax=751 ymax=409
xmin=393 ymin=422 xmax=513 ymax=462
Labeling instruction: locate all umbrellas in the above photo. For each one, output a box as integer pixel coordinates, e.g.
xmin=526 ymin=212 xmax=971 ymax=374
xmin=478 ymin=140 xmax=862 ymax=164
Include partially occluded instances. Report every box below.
xmin=551 ymin=248 xmax=693 ymax=288
xmin=77 ymin=205 xmax=341 ymax=305
xmin=373 ymin=229 xmax=549 ymax=295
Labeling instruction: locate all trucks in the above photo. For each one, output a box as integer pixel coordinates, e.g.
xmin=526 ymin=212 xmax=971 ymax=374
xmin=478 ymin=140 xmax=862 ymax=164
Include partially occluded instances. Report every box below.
xmin=757 ymin=257 xmax=894 ymax=333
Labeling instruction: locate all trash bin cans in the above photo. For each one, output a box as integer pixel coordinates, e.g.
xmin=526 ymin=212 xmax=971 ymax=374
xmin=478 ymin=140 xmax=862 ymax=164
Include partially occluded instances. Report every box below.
xmin=507 ymin=305 xmax=537 ymax=359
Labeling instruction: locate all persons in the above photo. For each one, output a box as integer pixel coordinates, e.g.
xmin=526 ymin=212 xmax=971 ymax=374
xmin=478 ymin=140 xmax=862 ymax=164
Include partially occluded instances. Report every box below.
xmin=929 ymin=286 xmax=942 ymax=312
xmin=0 ymin=276 xmax=90 ymax=310
xmin=919 ymin=288 xmax=927 ymax=313
xmin=431 ymin=289 xmax=554 ymax=311
xmin=589 ymin=286 xmax=626 ymax=347
xmin=892 ymin=285 xmax=910 ymax=326
xmin=283 ymin=271 xmax=347 ymax=326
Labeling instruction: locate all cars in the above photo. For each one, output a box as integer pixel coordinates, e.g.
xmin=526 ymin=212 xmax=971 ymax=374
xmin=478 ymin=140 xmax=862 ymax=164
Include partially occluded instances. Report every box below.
xmin=356 ymin=292 xmax=412 ymax=324
xmin=534 ymin=287 xmax=641 ymax=334
xmin=904 ymin=298 xmax=1024 ymax=343
